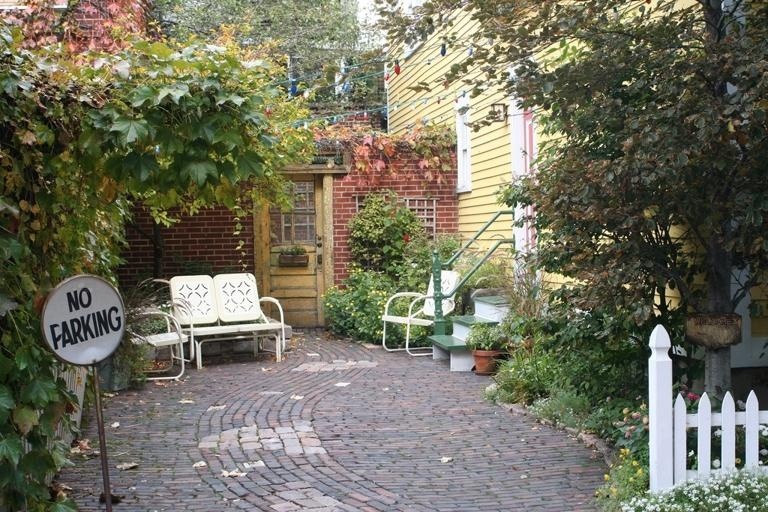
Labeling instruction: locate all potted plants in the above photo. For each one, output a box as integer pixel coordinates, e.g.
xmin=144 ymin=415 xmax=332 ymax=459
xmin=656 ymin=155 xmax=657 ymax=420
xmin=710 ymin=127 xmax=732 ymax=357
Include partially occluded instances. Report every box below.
xmin=279 ymin=245 xmax=308 ymax=267
xmin=465 ymin=323 xmax=510 ymax=374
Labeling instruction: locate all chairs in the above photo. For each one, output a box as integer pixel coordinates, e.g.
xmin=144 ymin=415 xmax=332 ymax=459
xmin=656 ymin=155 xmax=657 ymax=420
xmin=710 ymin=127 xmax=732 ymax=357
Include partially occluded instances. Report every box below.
xmin=121 ymin=302 xmax=191 ymax=385
xmin=381 ymin=268 xmax=458 ymax=357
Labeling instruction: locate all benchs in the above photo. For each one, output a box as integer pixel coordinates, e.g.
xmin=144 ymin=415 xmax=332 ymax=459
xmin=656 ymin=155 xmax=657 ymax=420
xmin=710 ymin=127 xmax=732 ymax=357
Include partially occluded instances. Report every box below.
xmin=169 ymin=273 xmax=285 ymax=383
